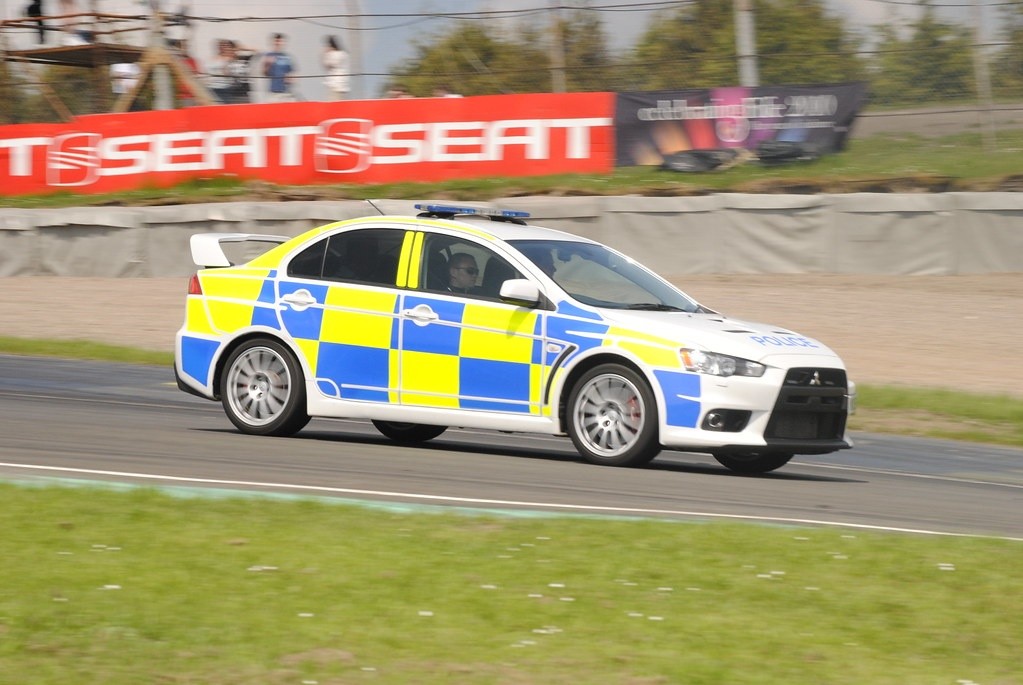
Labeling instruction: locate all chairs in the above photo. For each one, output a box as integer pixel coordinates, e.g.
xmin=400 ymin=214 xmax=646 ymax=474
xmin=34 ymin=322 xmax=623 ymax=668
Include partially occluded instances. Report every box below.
xmin=429 ymin=254 xmax=447 ymax=290
xmin=482 ymin=257 xmax=515 ymax=297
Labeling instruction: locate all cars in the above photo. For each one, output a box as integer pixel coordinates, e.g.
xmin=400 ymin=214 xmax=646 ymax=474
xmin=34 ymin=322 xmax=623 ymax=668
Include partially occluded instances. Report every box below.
xmin=174 ymin=204 xmax=855 ymax=476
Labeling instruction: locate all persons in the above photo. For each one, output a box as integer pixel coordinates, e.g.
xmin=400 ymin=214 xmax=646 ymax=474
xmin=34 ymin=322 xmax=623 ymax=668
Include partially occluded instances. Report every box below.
xmin=260 ymin=31 xmax=299 ymax=102
xmin=56 ymin=0 xmax=94 ymax=45
xmin=109 ymin=61 xmax=147 ymax=111
xmin=522 ymin=247 xmax=631 ymax=308
xmin=205 ymin=38 xmax=258 ymax=106
xmin=168 ymin=39 xmax=199 ymax=106
xmin=316 ymin=32 xmax=351 ymax=102
xmin=444 ymin=254 xmax=480 ymax=294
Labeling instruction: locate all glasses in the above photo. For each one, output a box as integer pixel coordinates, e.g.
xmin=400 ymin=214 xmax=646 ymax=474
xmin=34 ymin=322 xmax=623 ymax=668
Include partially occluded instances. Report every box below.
xmin=455 ymin=267 xmax=479 ymax=274
